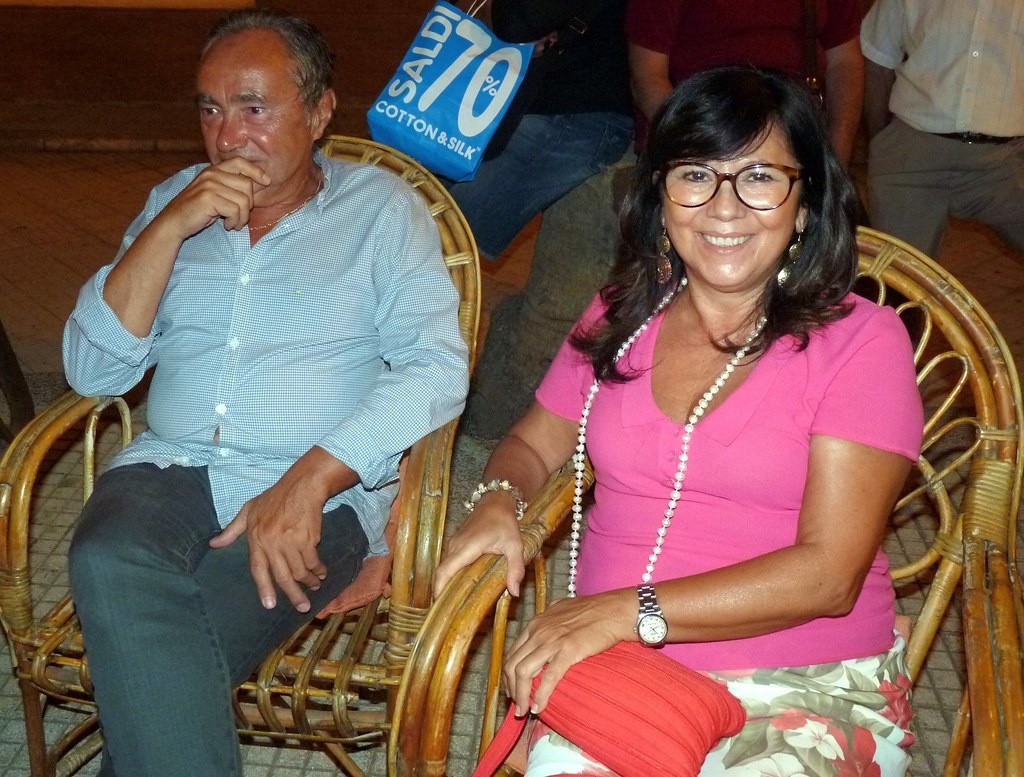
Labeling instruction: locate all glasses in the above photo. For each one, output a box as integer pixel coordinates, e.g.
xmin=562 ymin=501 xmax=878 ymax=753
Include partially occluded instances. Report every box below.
xmin=656 ymin=162 xmax=809 ymax=211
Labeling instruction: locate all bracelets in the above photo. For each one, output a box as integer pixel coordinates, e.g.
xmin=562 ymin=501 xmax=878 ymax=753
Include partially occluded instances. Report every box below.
xmin=464 ymin=479 xmax=528 ymax=520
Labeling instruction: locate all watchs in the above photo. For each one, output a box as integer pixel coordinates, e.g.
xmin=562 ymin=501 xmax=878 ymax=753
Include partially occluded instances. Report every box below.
xmin=634 ymin=583 xmax=668 ymax=649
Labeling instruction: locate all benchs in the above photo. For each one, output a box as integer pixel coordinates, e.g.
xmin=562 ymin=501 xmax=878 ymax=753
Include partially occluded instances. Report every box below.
xmin=1 ymin=98 xmax=1024 ymax=408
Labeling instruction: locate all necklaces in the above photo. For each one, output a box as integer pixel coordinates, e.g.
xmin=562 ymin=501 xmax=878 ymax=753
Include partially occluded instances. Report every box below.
xmin=567 ymin=277 xmax=768 ymax=598
xmin=247 ymin=169 xmax=324 ymax=231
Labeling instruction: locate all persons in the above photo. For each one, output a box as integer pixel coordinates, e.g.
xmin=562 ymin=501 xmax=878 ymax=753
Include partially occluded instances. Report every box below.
xmin=439 ymin=0 xmax=635 ymax=262
xmin=436 ymin=64 xmax=925 ymax=777
xmin=861 ymin=0 xmax=1024 ymax=259
xmin=626 ymin=0 xmax=870 ymax=225
xmin=64 ymin=9 xmax=468 ymax=777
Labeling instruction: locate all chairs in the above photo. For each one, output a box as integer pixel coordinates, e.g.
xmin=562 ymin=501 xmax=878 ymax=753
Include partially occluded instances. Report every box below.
xmin=387 ymin=226 xmax=1024 ymax=777
xmin=1 ymin=136 xmax=484 ymax=777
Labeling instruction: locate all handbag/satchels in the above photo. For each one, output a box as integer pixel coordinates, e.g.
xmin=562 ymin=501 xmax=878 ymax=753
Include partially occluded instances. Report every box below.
xmin=365 ymin=0 xmax=536 ymax=185
xmin=481 ymin=76 xmax=541 ymax=161
xmin=468 ymin=640 xmax=746 ymax=777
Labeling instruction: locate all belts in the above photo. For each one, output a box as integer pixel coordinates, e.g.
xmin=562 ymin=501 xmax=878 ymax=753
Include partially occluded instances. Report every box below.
xmin=930 ymin=131 xmax=1024 ymax=145
